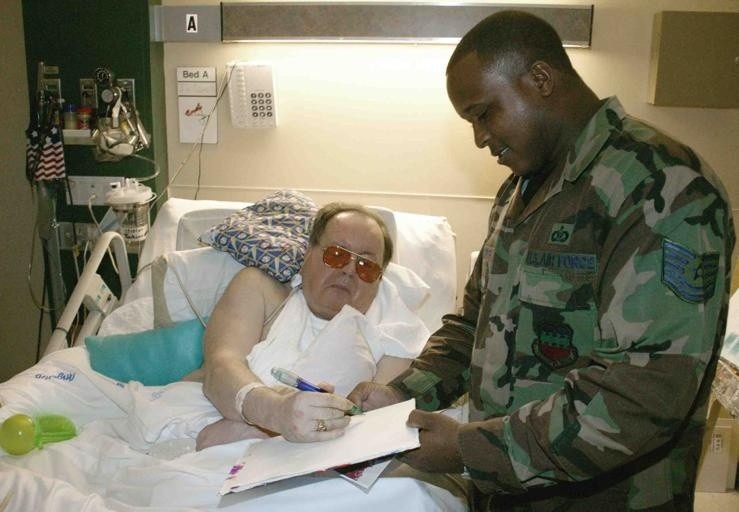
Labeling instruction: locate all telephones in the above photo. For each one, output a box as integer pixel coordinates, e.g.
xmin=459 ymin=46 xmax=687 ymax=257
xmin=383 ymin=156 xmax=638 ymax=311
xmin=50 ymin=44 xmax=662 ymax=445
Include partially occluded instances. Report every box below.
xmin=225 ymin=61 xmax=278 ymax=132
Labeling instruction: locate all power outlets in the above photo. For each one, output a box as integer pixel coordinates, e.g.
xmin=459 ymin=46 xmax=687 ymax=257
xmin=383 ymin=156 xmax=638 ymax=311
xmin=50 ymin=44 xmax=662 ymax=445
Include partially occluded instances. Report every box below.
xmin=63 ymin=175 xmax=127 ymax=208
xmin=57 ymin=221 xmax=100 ymax=252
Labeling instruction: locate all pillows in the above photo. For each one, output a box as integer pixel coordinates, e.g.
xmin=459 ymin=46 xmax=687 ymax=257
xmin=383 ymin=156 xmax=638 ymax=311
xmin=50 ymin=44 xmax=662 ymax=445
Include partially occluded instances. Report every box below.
xmin=197 ymin=188 xmax=323 ymax=284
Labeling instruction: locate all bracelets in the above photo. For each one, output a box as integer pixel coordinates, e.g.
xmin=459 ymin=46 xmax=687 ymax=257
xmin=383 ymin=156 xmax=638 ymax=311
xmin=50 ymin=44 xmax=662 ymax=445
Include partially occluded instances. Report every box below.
xmin=232 ymin=381 xmax=269 ymax=426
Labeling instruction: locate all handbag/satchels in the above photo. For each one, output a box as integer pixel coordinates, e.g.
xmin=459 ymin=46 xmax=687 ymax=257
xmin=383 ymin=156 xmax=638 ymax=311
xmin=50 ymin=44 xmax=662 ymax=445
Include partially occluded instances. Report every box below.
xmin=26 ymin=92 xmax=66 ymax=182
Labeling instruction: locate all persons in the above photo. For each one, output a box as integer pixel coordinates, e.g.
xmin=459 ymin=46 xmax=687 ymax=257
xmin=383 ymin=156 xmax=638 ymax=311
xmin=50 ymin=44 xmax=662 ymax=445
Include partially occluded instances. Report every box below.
xmin=179 ymin=202 xmax=429 ymax=452
xmin=350 ymin=10 xmax=737 ymax=511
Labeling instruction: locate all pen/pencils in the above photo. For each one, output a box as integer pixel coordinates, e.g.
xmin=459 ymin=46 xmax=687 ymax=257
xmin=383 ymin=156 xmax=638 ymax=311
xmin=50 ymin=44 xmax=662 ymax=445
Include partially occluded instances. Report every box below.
xmin=267 ymin=366 xmax=367 ymax=420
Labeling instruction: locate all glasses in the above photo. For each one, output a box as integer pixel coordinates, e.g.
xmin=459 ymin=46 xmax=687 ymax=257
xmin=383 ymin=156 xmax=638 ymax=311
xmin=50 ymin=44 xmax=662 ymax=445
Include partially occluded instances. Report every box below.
xmin=320 ymin=245 xmax=381 ymax=283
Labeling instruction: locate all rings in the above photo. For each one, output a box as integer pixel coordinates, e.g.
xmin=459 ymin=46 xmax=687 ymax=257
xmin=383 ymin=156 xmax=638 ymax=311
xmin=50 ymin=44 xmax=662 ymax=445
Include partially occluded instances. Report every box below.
xmin=314 ymin=419 xmax=328 ymax=432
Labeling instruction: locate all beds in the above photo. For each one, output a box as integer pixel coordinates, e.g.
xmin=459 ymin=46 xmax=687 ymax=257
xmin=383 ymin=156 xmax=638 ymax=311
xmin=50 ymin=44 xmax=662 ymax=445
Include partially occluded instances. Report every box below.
xmin=0 ymin=196 xmax=474 ymax=511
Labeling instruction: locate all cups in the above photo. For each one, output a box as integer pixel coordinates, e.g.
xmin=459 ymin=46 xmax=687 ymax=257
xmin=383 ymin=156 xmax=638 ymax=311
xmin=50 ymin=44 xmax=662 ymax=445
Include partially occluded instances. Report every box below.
xmin=109 ymin=185 xmax=152 ymax=241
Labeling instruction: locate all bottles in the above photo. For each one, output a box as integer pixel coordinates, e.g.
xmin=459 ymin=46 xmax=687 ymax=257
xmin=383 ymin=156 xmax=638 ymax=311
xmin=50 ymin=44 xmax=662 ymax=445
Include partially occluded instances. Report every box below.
xmin=63 ymin=105 xmax=77 ymax=130
